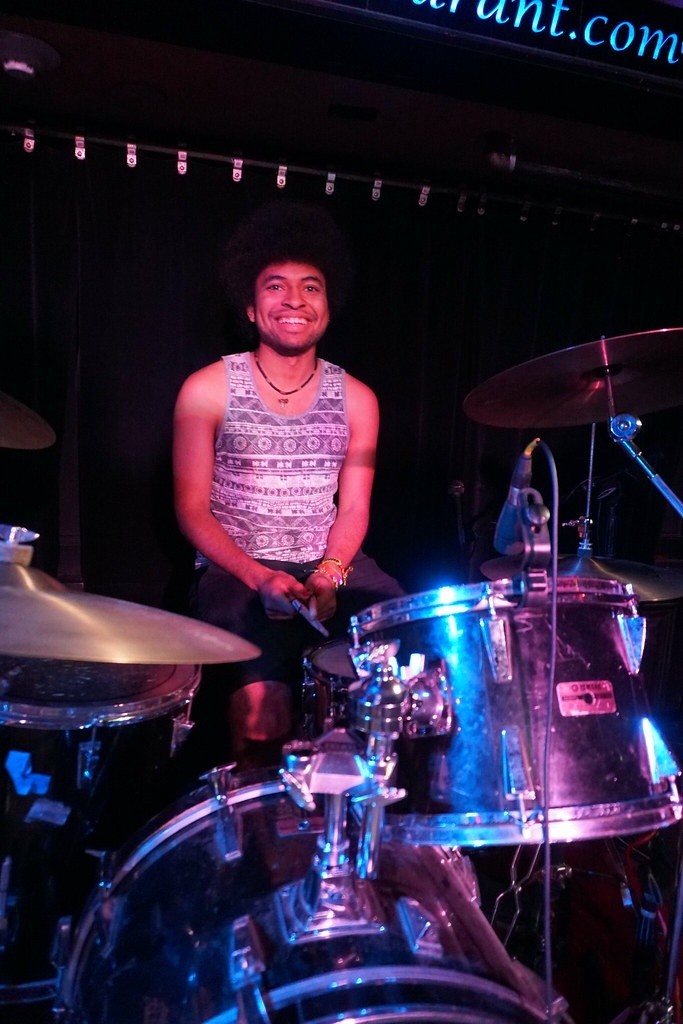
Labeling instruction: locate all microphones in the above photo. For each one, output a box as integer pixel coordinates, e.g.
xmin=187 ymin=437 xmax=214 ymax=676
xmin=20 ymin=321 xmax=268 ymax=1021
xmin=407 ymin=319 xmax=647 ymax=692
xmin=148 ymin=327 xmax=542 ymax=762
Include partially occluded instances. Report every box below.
xmin=493 ymin=439 xmax=540 ymax=556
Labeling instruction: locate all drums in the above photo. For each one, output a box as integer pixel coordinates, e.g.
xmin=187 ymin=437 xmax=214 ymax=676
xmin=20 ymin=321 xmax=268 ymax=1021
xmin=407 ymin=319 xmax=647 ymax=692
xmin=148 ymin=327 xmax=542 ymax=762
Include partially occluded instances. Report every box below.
xmin=346 ymin=577 xmax=683 ymax=856
xmin=0 ymin=650 xmax=207 ymax=1007
xmin=50 ymin=755 xmax=562 ymax=1024
xmin=299 ymin=630 xmax=361 ymax=739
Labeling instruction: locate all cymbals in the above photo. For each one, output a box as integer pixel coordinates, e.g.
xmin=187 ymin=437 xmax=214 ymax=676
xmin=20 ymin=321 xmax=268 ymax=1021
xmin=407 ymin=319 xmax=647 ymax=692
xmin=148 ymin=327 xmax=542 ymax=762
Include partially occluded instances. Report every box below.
xmin=478 ymin=547 xmax=682 ymax=604
xmin=459 ymin=325 xmax=683 ymax=429
xmin=0 ymin=560 xmax=263 ymax=669
xmin=0 ymin=388 xmax=58 ymax=451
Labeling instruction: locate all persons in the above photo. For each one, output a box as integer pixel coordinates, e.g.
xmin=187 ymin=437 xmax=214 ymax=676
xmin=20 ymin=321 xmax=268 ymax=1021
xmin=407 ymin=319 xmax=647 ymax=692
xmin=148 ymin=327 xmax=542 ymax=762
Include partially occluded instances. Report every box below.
xmin=171 ymin=199 xmax=408 ymax=774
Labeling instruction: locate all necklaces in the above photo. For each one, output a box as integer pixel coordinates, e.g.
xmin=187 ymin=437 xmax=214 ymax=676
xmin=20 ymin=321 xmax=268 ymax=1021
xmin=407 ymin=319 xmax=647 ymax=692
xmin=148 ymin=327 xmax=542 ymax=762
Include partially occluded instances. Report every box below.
xmin=255 ymin=351 xmax=318 ymax=407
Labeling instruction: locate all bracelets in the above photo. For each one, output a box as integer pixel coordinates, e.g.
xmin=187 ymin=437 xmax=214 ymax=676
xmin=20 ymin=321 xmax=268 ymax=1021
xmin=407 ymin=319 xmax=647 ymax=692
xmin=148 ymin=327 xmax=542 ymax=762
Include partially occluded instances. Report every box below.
xmin=314 ymin=558 xmax=353 ymax=592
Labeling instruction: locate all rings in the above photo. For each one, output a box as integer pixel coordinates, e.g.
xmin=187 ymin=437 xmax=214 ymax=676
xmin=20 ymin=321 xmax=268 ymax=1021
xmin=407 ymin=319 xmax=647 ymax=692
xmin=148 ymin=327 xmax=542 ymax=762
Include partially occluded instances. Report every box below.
xmin=329 ymin=608 xmax=333 ymax=618
